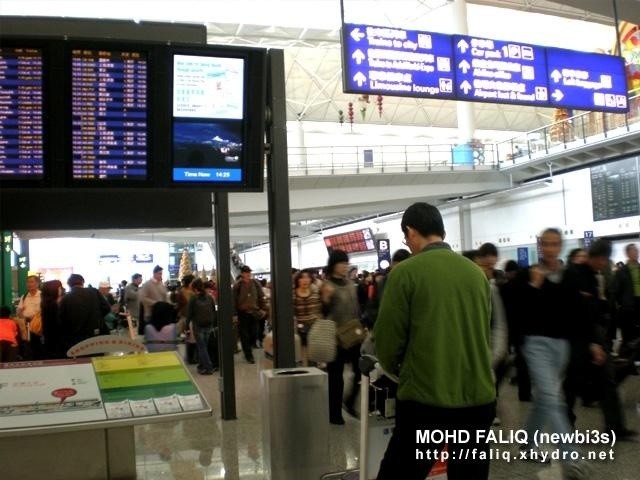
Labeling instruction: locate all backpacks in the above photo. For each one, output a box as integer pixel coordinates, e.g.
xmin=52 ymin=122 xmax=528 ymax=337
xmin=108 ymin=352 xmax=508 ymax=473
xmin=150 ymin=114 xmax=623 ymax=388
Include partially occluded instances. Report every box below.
xmin=193 ymin=299 xmax=215 ymax=328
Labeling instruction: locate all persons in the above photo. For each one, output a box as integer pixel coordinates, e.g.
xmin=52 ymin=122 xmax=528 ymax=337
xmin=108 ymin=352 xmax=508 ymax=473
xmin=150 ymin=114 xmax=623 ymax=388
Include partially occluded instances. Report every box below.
xmin=370 ymin=202 xmax=498 ymax=479
xmin=1 ymin=244 xmax=640 ymax=438
xmin=516 ymin=226 xmax=590 ymax=478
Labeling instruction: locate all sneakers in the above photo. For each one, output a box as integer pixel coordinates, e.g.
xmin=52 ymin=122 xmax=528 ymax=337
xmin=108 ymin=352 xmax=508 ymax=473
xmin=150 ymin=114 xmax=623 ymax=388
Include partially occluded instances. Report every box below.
xmin=516 ymin=444 xmax=552 ymax=464
xmin=492 ymin=416 xmax=501 ymax=425
xmin=561 ymin=459 xmax=594 ymax=480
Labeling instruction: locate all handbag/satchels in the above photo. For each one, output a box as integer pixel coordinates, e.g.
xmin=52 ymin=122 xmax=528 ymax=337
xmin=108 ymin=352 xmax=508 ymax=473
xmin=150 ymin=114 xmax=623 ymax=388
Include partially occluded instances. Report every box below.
xmin=306 ymin=317 xmax=339 ymax=363
xmin=336 ymin=318 xmax=366 ymax=350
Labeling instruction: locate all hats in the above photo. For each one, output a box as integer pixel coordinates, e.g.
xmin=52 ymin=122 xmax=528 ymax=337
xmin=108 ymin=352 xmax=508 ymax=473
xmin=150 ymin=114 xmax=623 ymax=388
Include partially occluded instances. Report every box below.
xmin=99 ymin=281 xmax=113 ymax=289
xmin=241 ymin=266 xmax=253 ymax=273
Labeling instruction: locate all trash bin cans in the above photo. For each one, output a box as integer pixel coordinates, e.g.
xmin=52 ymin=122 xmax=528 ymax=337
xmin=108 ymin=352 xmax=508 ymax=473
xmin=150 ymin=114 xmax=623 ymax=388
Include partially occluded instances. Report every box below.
xmin=260 ymin=367 xmax=331 ymax=479
